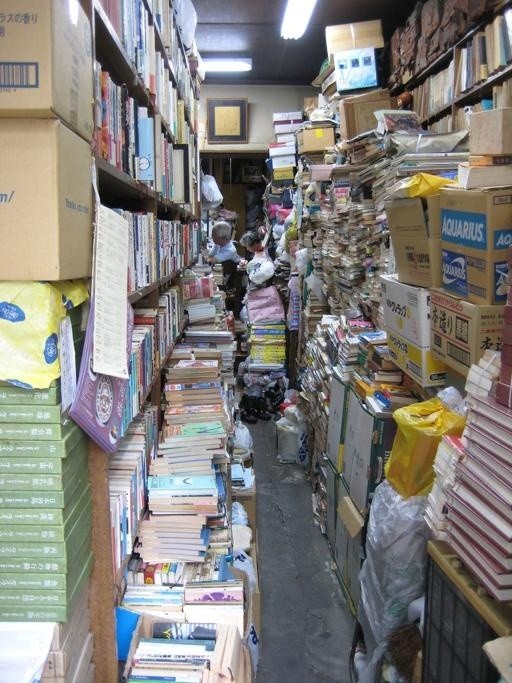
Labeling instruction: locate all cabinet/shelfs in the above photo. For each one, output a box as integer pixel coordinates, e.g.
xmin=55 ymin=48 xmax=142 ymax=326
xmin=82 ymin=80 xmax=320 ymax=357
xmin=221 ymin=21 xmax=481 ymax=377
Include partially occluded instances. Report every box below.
xmin=79 ymin=1 xmax=203 ymax=683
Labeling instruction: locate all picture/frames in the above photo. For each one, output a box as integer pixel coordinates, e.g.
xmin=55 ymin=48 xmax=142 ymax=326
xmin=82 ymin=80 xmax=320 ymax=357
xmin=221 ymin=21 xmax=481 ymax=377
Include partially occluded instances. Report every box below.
xmin=206 ymin=97 xmax=248 ymax=144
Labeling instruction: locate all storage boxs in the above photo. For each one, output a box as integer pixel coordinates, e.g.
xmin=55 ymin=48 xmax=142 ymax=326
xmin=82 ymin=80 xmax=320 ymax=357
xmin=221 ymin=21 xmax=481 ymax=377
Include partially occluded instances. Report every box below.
xmin=229 ymin=467 xmax=261 ymax=662
xmin=0 ymin=0 xmax=99 ymax=284
xmin=269 ymin=19 xmax=512 ymax=683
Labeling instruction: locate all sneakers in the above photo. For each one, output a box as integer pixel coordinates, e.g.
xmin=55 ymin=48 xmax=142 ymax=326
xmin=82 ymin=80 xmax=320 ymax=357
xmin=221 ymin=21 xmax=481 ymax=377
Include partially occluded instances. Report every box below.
xmin=240 ymin=414 xmax=257 ymax=423
xmin=251 ymin=408 xmax=273 ymax=420
